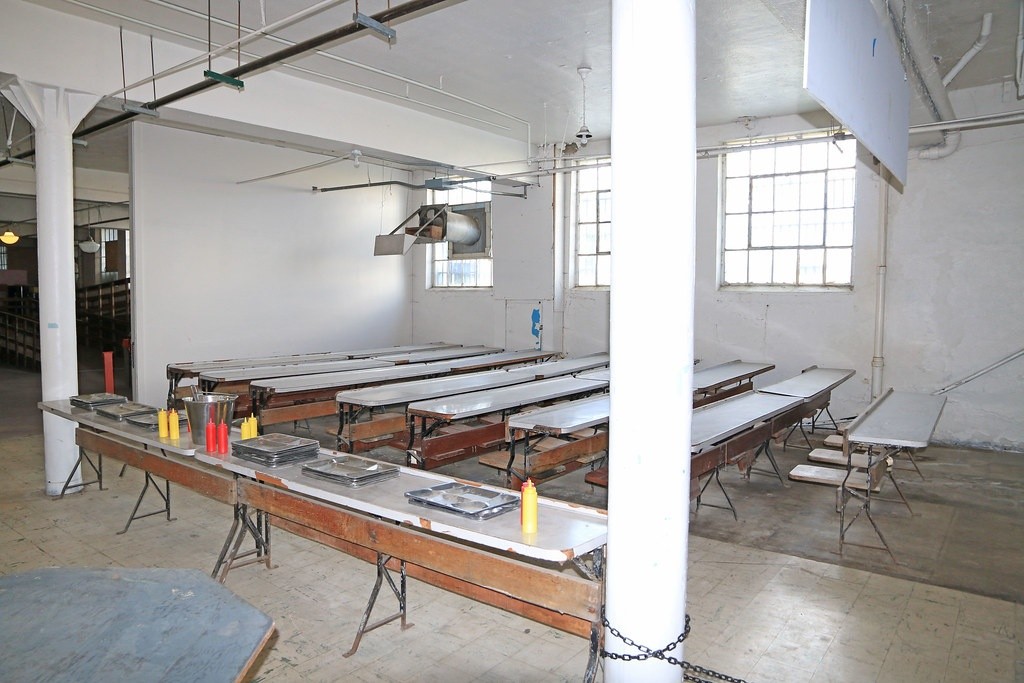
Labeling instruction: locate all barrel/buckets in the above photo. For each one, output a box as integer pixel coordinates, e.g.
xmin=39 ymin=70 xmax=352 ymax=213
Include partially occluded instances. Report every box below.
xmin=182 ymin=394 xmax=239 ymax=445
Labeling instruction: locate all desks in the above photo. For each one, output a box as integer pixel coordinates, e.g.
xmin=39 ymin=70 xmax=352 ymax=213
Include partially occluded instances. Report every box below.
xmin=164 ymin=341 xmax=857 ymax=482
xmin=838 ymin=386 xmax=948 ymax=567
xmin=0 ymin=565 xmax=279 ymax=683
xmin=37 ymin=390 xmax=608 ymax=682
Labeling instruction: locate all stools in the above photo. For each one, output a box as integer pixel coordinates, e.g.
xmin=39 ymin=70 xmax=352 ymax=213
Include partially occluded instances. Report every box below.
xmin=324 ymin=397 xmax=924 ymax=564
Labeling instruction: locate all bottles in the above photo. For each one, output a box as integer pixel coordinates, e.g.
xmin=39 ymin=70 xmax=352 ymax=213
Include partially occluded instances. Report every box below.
xmin=523 ymin=480 xmax=537 ymax=535
xmin=217 ymin=418 xmax=228 ymax=454
xmin=205 ymin=418 xmax=216 ymax=452
xmin=157 ymin=408 xmax=168 ymax=438
xmin=520 ymin=477 xmax=535 ymax=526
xmin=248 ymin=413 xmax=257 ymax=438
xmin=168 ymin=407 xmax=179 ymax=439
xmin=241 ymin=417 xmax=251 ymax=440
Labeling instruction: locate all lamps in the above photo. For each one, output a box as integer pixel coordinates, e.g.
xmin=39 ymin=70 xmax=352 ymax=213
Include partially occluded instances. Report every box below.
xmin=351 ymin=150 xmax=362 ymax=169
xmin=0 ymin=221 xmax=20 ymax=245
xmin=577 ymin=66 xmax=593 ymax=145
xmin=78 ymin=202 xmax=100 ymax=253
